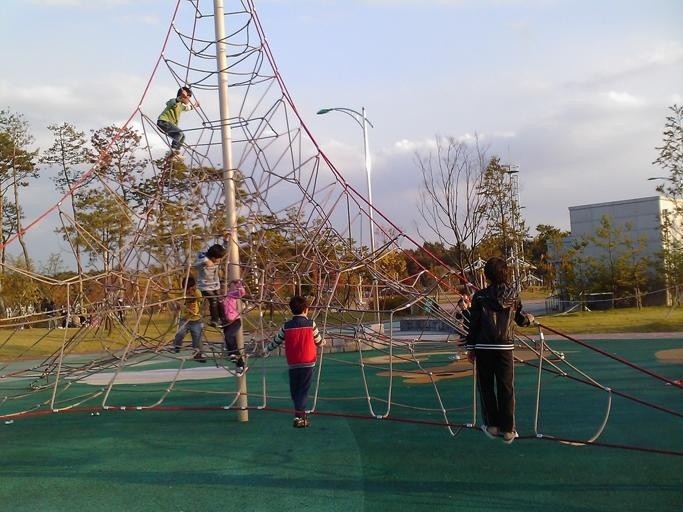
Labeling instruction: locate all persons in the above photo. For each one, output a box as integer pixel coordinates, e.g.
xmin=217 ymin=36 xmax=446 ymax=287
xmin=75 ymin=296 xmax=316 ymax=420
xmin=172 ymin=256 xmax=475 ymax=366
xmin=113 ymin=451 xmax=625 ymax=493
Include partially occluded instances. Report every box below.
xmin=157 ymin=87 xmax=200 ymax=163
xmin=6 ymin=296 xmax=124 ymax=330
xmin=168 ymin=244 xmax=249 ymax=377
xmin=467 ymin=258 xmax=535 ymax=444
xmin=417 ymin=291 xmax=441 ymax=316
xmin=261 ymin=296 xmax=326 ymax=427
xmin=456 ymin=292 xmax=472 ymax=346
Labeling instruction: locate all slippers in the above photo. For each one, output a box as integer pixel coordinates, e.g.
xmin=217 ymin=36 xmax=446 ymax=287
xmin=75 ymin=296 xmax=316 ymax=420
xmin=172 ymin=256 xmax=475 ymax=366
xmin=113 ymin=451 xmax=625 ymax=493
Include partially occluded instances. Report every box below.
xmin=503 ymin=430 xmax=519 ymax=444
xmin=481 ymin=424 xmax=499 ymax=440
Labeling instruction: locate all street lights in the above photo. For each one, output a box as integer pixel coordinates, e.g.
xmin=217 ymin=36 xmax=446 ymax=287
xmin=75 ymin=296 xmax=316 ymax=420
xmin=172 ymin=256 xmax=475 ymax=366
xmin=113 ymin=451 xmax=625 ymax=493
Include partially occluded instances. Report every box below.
xmin=317 ymin=104 xmax=381 ymax=324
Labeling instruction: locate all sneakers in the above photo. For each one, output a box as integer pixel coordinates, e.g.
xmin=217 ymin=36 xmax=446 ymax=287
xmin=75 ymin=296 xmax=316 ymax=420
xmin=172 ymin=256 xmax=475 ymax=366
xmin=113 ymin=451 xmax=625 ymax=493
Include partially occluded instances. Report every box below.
xmin=229 ymin=354 xmax=248 ymax=377
xmin=194 ymin=354 xmax=207 ymax=363
xmin=208 ymin=321 xmax=217 ymax=327
xmin=449 ymin=355 xmax=461 ymax=360
xmin=294 ymin=416 xmax=310 ymax=428
